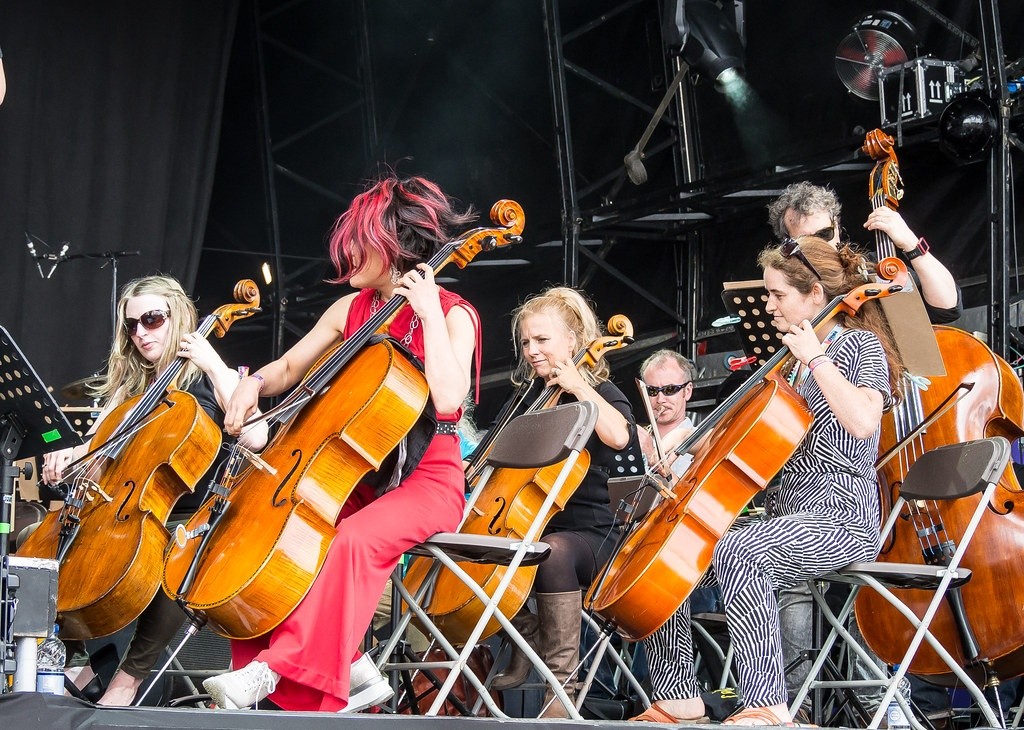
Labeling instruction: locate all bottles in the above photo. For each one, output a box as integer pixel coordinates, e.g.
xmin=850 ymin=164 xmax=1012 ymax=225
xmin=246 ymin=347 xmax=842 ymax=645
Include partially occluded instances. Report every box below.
xmin=37 ymin=623 xmax=66 ymax=697
xmin=888 ymin=663 xmax=911 ymax=730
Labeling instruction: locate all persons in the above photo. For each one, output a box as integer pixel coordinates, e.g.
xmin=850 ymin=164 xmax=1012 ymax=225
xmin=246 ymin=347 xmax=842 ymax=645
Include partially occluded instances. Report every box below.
xmin=905 ymin=671 xmax=1022 ymax=729
xmin=625 ymin=234 xmax=894 ymax=728
xmin=201 ymin=173 xmax=484 ymax=714
xmin=356 ymin=391 xmax=482 ymax=688
xmin=579 ymin=347 xmax=724 ymax=699
xmin=767 ymin=180 xmax=963 ymax=728
xmin=37 ymin=273 xmax=269 ymax=706
xmin=459 ymin=287 xmax=646 ymax=720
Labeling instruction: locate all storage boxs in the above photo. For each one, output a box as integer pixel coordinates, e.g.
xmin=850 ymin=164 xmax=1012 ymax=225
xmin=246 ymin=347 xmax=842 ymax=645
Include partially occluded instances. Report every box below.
xmin=878 ymin=59 xmax=956 ymax=125
xmin=8 ymin=557 xmax=59 ymax=637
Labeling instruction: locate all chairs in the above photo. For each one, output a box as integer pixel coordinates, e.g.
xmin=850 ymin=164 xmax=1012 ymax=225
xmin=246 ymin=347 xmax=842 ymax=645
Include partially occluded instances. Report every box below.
xmin=9 ymin=401 xmax=1012 ymax=730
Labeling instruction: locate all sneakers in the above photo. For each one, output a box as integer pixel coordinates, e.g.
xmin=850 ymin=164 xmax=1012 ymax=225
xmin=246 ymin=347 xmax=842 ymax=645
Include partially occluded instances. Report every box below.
xmin=337 ymin=651 xmax=396 ymax=713
xmin=201 ymin=661 xmax=282 ymax=710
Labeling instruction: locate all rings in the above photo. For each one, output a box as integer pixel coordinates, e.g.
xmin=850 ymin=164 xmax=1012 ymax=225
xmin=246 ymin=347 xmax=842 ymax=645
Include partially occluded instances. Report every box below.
xmin=554 ymin=368 xmax=559 ymax=375
xmin=42 ymin=463 xmax=47 ymax=468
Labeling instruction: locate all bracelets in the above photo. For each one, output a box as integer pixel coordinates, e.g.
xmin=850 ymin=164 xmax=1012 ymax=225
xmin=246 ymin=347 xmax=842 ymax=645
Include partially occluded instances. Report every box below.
xmin=808 ymin=354 xmax=833 ymax=371
xmin=237 ymin=366 xmax=265 ymax=392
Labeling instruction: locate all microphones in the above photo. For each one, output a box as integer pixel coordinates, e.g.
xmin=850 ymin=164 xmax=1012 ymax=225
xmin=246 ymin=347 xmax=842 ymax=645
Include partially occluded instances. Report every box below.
xmin=26 ymin=238 xmax=44 ymax=278
xmin=48 ymin=242 xmax=70 ymax=278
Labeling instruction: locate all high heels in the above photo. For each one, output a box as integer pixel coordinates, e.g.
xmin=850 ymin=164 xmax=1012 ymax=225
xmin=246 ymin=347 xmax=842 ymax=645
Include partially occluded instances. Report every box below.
xmin=78 ymin=676 xmax=104 ymax=701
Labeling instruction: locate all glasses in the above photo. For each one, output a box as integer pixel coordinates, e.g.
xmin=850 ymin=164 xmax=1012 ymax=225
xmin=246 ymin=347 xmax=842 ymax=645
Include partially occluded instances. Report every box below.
xmin=780 ymin=238 xmax=822 ymax=281
xmin=646 ymin=380 xmax=694 ymax=397
xmin=124 ymin=308 xmax=171 ymax=336
xmin=810 ymin=212 xmax=836 ymax=242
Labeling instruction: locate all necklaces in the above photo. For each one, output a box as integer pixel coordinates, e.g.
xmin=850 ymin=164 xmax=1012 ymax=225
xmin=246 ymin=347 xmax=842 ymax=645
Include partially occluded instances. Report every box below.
xmin=368 ymin=289 xmax=420 ymax=348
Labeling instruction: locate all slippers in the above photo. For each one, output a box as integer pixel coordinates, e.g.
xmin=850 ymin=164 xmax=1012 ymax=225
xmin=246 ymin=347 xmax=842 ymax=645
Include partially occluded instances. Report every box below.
xmin=720 ymin=708 xmax=793 ymax=726
xmin=627 ymin=702 xmax=711 ymax=723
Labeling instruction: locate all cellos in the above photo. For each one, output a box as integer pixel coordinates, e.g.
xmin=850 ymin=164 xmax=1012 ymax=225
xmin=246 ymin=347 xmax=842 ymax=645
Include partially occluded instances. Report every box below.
xmin=853 ymin=124 xmax=1024 ymax=721
xmin=396 ymin=311 xmax=635 ymax=709
xmin=133 ymin=196 xmax=528 ymax=707
xmin=535 ymin=252 xmax=909 ymax=721
xmin=14 ymin=275 xmax=264 ymax=644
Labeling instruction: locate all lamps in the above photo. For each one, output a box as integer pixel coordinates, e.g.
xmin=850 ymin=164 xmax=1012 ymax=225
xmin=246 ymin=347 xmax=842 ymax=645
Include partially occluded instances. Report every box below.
xmin=661 ymin=0 xmax=747 ymax=81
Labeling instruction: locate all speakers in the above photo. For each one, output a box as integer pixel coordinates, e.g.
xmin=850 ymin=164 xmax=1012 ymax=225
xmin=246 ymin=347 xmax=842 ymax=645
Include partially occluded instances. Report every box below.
xmin=159 ymin=511 xmax=234 ymax=708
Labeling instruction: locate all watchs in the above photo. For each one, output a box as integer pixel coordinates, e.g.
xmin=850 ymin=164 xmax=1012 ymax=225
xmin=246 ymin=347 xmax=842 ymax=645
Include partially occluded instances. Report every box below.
xmin=901 ymin=237 xmax=930 ymax=260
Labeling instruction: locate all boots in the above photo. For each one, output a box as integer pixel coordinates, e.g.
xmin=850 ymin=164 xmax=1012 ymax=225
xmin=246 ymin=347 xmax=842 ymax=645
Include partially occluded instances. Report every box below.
xmin=490 ymin=608 xmax=538 ymax=691
xmin=536 ymin=589 xmax=582 ymax=718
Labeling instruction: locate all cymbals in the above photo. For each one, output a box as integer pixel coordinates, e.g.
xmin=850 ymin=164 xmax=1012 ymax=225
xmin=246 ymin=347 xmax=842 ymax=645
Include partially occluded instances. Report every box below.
xmin=61 ymin=375 xmax=106 ymax=400
